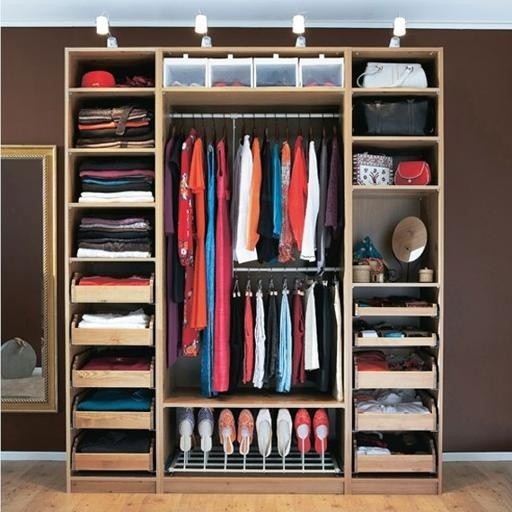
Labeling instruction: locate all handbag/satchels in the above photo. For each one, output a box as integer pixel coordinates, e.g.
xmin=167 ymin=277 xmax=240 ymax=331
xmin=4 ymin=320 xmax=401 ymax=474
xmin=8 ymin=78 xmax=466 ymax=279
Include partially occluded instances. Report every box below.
xmin=353 ymin=150 xmax=395 ymax=188
xmin=364 ymin=98 xmax=437 ymax=136
xmin=356 ymin=60 xmax=429 ymax=90
xmin=394 ymin=159 xmax=433 ymax=185
xmin=354 ymin=235 xmax=391 ymax=283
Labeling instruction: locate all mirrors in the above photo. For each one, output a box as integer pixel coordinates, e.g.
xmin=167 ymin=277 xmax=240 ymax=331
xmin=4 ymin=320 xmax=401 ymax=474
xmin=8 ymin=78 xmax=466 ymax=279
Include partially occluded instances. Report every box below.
xmin=2 ymin=144 xmax=59 ymax=414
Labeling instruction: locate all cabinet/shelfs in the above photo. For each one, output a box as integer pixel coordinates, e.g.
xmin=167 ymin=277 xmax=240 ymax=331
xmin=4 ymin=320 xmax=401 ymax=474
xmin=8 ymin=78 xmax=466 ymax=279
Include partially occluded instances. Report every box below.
xmin=63 ymin=47 xmax=443 ymax=494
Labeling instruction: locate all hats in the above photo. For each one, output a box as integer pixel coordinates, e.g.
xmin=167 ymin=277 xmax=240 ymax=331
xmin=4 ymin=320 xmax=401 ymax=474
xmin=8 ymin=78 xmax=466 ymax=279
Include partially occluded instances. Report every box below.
xmin=81 ymin=71 xmax=129 ymax=87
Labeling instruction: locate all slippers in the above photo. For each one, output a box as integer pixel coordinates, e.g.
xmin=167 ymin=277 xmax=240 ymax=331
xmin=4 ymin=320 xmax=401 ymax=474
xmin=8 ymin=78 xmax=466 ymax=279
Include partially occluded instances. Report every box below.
xmin=218 ymin=409 xmax=237 ymax=455
xmin=197 ymin=408 xmax=213 ymax=454
xmin=237 ymin=410 xmax=254 ymax=455
xmin=178 ymin=407 xmax=195 ymax=453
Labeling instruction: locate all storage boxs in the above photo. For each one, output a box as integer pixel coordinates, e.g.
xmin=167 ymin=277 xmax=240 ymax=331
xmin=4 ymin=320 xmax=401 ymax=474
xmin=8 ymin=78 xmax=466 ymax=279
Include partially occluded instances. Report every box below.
xmin=163 ymin=55 xmax=344 ymax=89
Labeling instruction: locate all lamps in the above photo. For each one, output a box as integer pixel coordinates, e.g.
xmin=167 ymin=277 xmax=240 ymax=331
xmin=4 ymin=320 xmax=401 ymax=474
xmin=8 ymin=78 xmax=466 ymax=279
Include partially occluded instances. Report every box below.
xmin=96 ymin=11 xmax=120 ymax=47
xmin=292 ymin=9 xmax=306 ymax=47
xmin=194 ymin=8 xmax=213 ymax=47
xmin=388 ymin=10 xmax=407 ymax=47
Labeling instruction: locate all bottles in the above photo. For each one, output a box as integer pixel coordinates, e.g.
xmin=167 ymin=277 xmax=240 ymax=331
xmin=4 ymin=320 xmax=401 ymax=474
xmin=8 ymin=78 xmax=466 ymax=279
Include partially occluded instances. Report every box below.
xmin=419 ymin=267 xmax=433 ymax=282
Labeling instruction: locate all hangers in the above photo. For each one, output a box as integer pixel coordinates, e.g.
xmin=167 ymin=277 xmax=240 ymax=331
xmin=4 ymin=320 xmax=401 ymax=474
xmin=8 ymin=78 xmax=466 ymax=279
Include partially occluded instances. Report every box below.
xmin=169 ymin=110 xmax=338 ymax=152
xmin=232 ymin=264 xmax=341 ymax=297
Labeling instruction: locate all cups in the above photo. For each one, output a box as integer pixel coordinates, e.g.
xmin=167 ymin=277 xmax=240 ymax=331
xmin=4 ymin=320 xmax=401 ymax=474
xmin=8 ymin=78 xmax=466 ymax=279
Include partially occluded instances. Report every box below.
xmin=353 ymin=265 xmax=371 ymax=283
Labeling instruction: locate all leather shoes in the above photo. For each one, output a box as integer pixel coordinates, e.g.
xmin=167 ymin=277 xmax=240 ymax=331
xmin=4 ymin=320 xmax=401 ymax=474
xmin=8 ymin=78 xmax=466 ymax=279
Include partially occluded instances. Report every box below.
xmin=276 ymin=410 xmax=290 ymax=455
xmin=313 ymin=409 xmax=327 ymax=454
xmin=294 ymin=410 xmax=312 ymax=453
xmin=256 ymin=410 xmax=274 ymax=458
